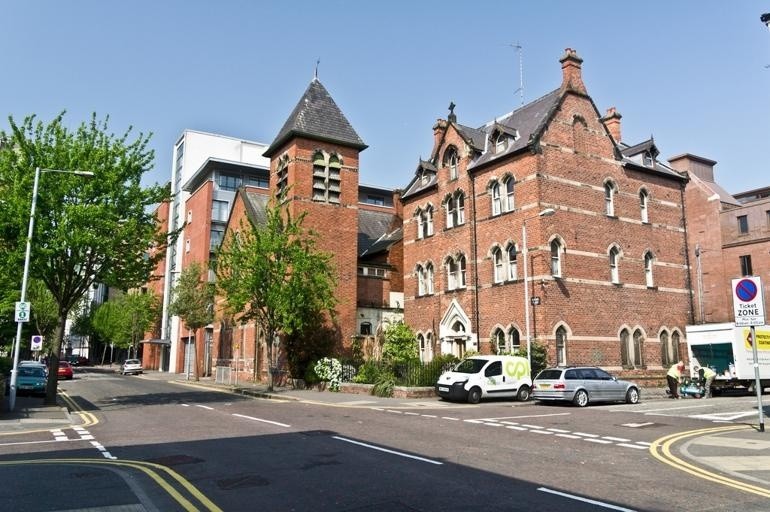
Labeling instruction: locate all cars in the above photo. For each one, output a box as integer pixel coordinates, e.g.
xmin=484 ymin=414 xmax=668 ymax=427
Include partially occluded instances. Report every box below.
xmin=5 ymin=355 xmax=87 ymax=398
xmin=121 ymin=359 xmax=144 ymax=375
xmin=531 ymin=367 xmax=641 ymax=407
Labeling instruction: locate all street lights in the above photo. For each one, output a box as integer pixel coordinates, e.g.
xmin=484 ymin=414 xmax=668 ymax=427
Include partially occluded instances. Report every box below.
xmin=520 ymin=207 xmax=556 ymax=369
xmin=8 ymin=167 xmax=96 ymax=414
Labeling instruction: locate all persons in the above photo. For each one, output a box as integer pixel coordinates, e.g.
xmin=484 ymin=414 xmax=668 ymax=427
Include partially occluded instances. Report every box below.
xmin=666 ymin=359 xmax=686 ymax=399
xmin=694 ymin=364 xmax=717 ymax=399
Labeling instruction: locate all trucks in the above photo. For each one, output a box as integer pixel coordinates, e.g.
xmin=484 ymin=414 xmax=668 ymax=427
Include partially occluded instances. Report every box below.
xmin=684 ymin=322 xmax=770 ymax=396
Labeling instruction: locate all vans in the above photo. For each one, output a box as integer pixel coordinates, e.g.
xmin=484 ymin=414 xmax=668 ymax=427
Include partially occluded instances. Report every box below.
xmin=435 ymin=355 xmax=532 ymax=403
